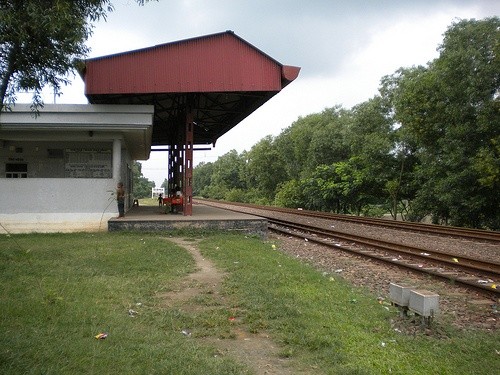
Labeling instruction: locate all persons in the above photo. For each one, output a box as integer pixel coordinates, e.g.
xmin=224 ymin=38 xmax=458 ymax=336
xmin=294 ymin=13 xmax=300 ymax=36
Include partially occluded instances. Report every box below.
xmin=116 ymin=183 xmax=125 ymax=217
xmin=158 ymin=194 xmax=163 ymax=207
xmin=168 ymin=185 xmax=182 ymax=214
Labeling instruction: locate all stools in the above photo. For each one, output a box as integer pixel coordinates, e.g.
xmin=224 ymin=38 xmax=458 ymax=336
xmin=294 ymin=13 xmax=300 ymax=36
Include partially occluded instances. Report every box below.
xmin=172 ymin=196 xmax=183 ymax=214
xmin=164 ymin=198 xmax=170 ymax=204
